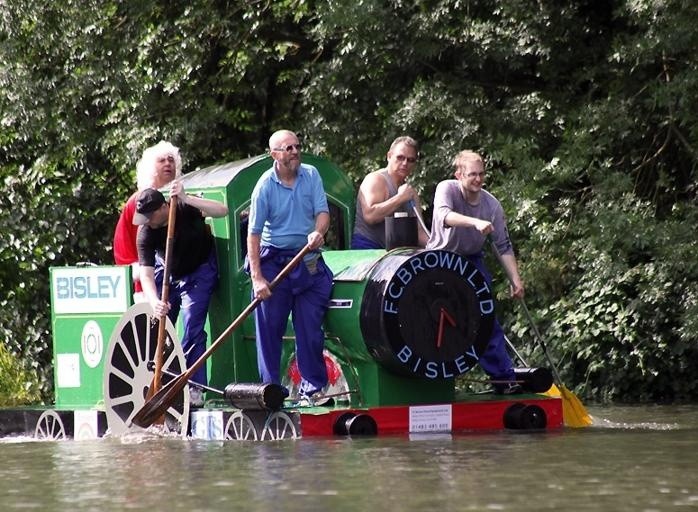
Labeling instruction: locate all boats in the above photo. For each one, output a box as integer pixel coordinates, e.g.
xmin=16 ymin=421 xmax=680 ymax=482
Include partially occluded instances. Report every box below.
xmin=1 ymin=150 xmax=574 ymax=443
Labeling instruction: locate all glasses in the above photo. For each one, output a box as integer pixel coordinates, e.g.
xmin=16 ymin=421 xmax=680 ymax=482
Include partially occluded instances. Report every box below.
xmin=274 ymin=144 xmax=301 ymax=151
xmin=397 ymin=155 xmax=415 ymax=162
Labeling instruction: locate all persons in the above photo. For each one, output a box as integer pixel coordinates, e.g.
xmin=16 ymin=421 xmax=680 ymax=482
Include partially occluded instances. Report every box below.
xmin=245 ymin=128 xmax=336 ymax=406
xmin=111 ymin=138 xmax=186 ymax=304
xmin=133 ymin=177 xmax=231 ymax=407
xmin=348 ymin=135 xmax=431 ymax=251
xmin=426 ymin=149 xmax=528 ymax=397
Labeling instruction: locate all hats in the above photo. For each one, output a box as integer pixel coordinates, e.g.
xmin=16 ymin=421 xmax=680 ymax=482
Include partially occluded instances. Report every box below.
xmin=132 ymin=188 xmax=165 ymax=225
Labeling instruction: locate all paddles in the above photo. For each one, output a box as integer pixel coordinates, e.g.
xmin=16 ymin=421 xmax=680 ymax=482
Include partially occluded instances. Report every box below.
xmin=486 ymin=233 xmax=592 ymax=427
xmin=145 ymin=195 xmax=177 ymax=427
xmin=408 ymin=198 xmax=561 ymax=397
xmin=131 ymin=244 xmax=313 ymax=427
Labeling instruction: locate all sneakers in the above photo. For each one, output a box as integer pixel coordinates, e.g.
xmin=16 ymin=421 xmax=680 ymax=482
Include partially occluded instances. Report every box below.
xmin=301 ymin=392 xmax=335 ymax=407
xmin=189 ymin=386 xmax=204 ymax=407
xmin=495 ymin=382 xmax=521 ymax=394
